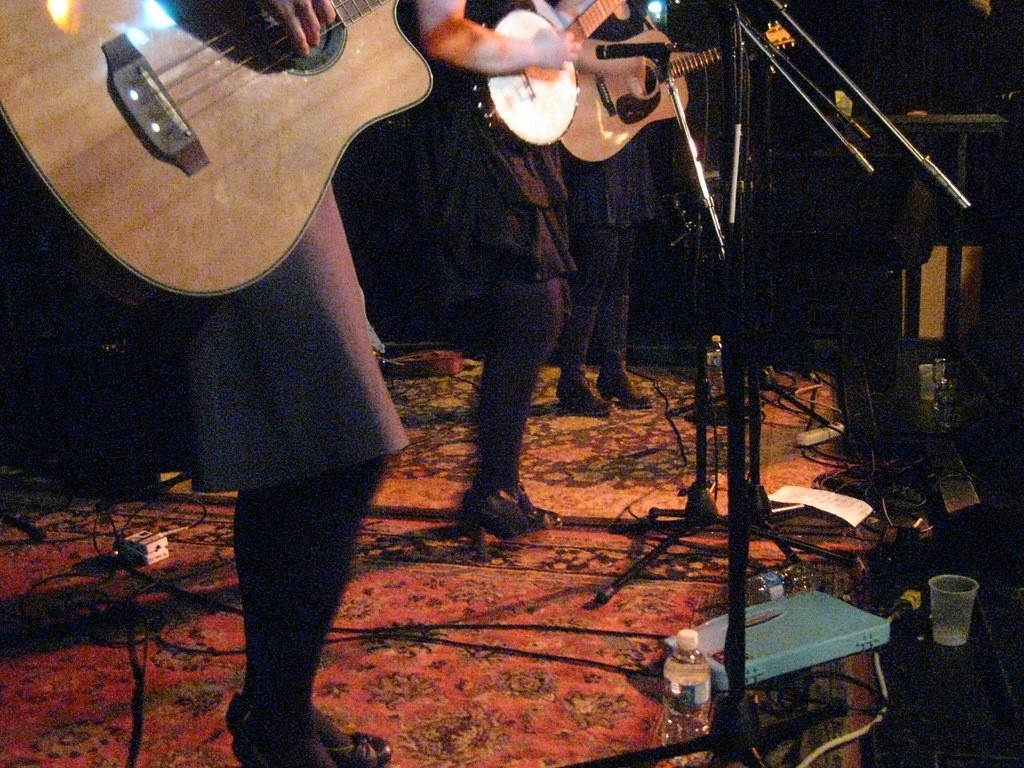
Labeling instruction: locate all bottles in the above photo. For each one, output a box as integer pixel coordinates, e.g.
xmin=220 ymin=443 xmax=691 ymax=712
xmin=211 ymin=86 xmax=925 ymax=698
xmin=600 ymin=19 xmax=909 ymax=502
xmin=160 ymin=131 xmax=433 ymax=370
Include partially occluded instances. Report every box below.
xmin=662 ymin=629 xmax=711 ymax=767
xmin=707 ymin=336 xmax=724 ymax=396
xmin=747 ymin=562 xmax=823 ymax=606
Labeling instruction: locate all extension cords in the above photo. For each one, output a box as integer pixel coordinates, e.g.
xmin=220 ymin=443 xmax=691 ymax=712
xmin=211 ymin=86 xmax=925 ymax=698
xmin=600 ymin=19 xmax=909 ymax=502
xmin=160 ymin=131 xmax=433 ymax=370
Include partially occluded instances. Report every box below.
xmin=796 ymin=422 xmax=845 ymax=446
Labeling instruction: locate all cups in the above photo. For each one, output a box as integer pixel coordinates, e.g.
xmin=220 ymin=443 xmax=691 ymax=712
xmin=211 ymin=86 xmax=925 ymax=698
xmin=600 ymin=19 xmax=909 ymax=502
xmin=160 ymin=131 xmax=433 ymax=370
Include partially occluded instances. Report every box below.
xmin=919 ymin=358 xmax=947 ymax=400
xmin=928 ymin=575 xmax=979 ymax=646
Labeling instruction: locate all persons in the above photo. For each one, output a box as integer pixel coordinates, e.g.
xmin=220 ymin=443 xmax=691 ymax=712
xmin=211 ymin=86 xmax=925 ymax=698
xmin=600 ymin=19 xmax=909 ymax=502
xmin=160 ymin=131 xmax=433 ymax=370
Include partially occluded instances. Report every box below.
xmin=556 ymin=0 xmax=662 ymax=417
xmin=386 ymin=0 xmax=582 ymax=538
xmin=83 ymin=0 xmax=407 ymax=768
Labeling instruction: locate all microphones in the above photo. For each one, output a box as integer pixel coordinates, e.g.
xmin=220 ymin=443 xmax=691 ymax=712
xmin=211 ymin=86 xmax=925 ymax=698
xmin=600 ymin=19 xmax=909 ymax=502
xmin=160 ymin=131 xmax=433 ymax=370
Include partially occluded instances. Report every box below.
xmin=595 ymin=42 xmax=693 ymax=60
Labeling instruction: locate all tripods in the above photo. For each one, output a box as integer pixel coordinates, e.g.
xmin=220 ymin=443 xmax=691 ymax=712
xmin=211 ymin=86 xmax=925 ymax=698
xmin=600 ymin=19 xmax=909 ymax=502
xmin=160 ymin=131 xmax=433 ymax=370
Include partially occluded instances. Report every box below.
xmin=555 ymin=0 xmax=971 ymax=768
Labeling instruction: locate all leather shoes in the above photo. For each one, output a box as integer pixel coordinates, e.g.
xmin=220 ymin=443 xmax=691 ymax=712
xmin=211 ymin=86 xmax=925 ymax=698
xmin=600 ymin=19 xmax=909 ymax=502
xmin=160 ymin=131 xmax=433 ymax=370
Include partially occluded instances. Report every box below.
xmin=226 ymin=693 xmax=393 ymax=768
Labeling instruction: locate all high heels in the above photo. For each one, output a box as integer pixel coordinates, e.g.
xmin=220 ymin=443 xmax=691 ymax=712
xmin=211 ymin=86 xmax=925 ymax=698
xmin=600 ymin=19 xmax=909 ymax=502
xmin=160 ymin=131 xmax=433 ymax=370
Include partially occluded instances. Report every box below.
xmin=460 ymin=488 xmax=538 ymax=545
xmin=596 ymin=374 xmax=652 ymax=409
xmin=504 ymin=481 xmax=562 ymax=531
xmin=558 ymin=385 xmax=612 ymax=416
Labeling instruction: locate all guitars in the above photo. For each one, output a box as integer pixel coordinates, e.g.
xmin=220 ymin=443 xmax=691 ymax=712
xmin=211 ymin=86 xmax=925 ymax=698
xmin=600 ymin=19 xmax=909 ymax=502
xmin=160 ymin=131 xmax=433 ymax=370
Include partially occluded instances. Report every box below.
xmin=557 ymin=19 xmax=799 ymax=164
xmin=2 ymin=0 xmax=438 ymax=304
xmin=465 ymin=0 xmax=628 ymax=152
